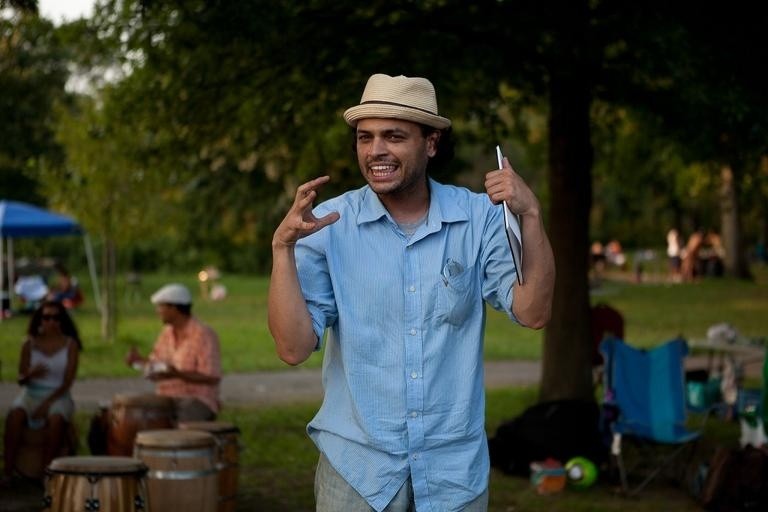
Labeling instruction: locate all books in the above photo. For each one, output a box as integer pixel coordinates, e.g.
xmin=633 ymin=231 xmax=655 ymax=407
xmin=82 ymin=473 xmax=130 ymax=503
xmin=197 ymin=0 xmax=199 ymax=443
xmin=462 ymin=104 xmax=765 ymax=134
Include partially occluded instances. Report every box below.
xmin=496 ymin=143 xmax=524 ymax=286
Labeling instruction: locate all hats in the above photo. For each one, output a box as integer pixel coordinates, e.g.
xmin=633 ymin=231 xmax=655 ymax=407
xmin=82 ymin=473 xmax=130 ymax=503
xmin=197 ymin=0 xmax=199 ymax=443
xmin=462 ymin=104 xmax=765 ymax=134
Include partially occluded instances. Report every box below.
xmin=149 ymin=282 xmax=193 ymax=307
xmin=343 ymin=73 xmax=453 ymax=133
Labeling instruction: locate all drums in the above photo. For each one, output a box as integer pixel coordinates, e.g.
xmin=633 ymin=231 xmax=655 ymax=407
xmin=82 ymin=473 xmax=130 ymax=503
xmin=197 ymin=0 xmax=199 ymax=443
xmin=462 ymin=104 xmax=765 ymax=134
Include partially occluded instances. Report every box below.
xmin=43 ymin=455 xmax=148 ymax=512
xmin=180 ymin=420 xmax=240 ymax=511
xmin=15 ymin=415 xmax=79 ymax=481
xmin=133 ymin=428 xmax=219 ymax=512
xmin=106 ymin=395 xmax=175 ymax=456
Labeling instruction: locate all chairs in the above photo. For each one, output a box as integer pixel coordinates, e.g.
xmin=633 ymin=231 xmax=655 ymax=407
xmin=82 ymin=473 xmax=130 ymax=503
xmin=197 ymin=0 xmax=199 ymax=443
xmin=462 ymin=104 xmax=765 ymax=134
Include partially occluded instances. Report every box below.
xmin=595 ymin=334 xmax=724 ymax=500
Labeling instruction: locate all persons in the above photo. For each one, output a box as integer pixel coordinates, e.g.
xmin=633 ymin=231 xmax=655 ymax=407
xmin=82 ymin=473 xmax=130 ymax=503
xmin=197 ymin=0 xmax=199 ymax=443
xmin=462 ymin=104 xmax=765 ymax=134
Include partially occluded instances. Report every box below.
xmin=269 ymin=73 xmax=556 ymax=510
xmin=594 ymin=218 xmax=728 ymax=282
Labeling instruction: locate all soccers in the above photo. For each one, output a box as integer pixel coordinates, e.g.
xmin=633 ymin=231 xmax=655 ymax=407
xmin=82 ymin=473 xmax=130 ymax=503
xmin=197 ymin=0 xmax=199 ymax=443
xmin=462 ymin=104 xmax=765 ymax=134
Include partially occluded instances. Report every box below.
xmin=563 ymin=456 xmax=600 ymax=488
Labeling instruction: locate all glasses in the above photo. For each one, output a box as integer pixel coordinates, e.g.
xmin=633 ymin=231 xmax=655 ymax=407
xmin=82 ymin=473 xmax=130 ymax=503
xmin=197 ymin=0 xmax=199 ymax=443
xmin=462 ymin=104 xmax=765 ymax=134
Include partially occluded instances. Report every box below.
xmin=42 ymin=313 xmax=60 ymax=320
